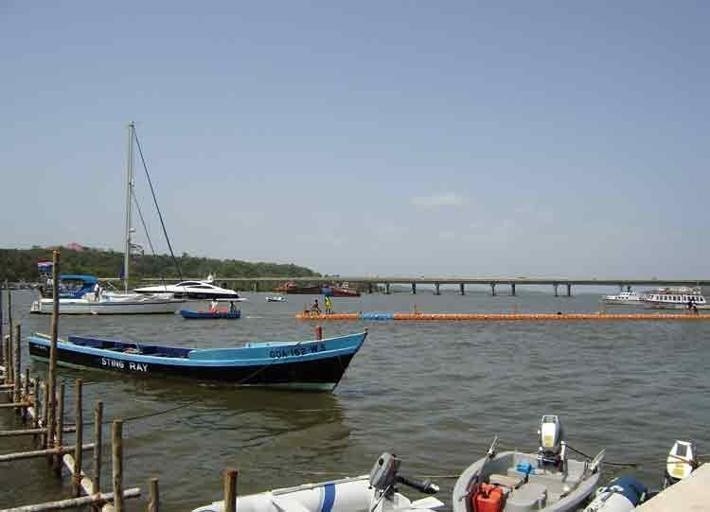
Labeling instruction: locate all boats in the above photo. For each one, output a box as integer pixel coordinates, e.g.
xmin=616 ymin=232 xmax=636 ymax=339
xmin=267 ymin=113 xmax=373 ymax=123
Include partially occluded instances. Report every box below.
xmin=173 ymin=303 xmax=242 ymax=321
xmin=262 ymin=277 xmax=361 ymax=305
xmin=598 ymin=287 xmax=649 ymax=308
xmin=133 ymin=277 xmax=246 ymax=303
xmin=452 ymin=410 xmax=606 ymax=512
xmin=191 ymin=453 xmax=444 ymax=512
xmin=638 ymin=285 xmax=709 ymax=309
xmin=585 ymin=433 xmax=710 ymax=512
xmin=25 ymin=327 xmax=369 ymax=393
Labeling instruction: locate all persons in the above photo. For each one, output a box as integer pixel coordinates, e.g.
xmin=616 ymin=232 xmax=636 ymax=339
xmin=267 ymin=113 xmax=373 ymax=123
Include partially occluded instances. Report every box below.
xmin=324 ymin=293 xmax=334 ymax=313
xmin=210 ymin=295 xmax=218 ymax=311
xmin=92 ymin=280 xmax=101 ymax=301
xmin=686 ymin=299 xmax=694 ymax=320
xmin=311 ymin=299 xmax=322 ymax=314
xmin=206 ymin=270 xmax=218 ymax=286
xmin=692 ymin=305 xmax=700 ymax=320
xmin=228 ymin=300 xmax=237 ymax=311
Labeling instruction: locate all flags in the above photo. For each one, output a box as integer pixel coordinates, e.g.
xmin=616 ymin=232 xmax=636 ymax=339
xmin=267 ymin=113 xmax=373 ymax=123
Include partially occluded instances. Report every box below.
xmin=35 ymin=255 xmax=54 ymax=273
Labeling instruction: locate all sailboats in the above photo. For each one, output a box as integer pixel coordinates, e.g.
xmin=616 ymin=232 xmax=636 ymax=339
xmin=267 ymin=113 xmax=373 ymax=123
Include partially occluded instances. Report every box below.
xmin=26 ymin=117 xmax=190 ymax=316
